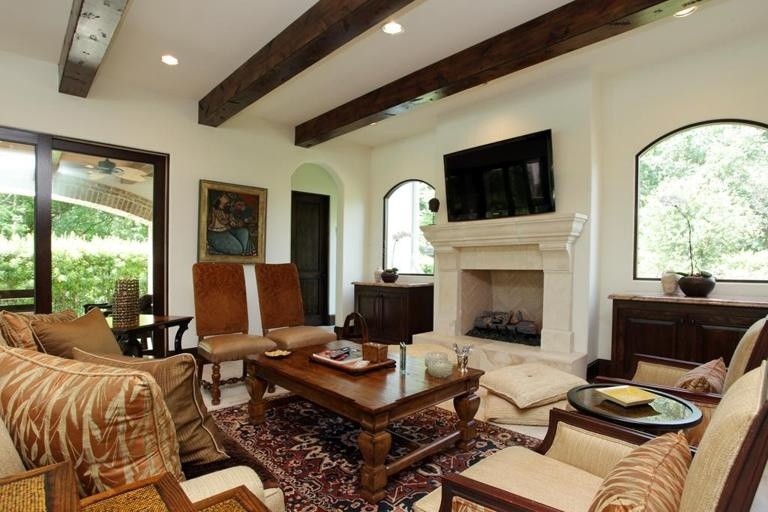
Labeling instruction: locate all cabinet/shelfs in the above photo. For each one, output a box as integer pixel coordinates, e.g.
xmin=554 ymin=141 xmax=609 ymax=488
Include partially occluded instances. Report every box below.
xmin=349 ymin=280 xmax=433 ymax=346
xmin=605 ymin=292 xmax=766 ymax=387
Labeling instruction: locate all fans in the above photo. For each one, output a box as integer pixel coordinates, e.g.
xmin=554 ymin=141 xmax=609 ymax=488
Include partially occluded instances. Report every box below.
xmin=71 ymin=154 xmax=147 ymax=187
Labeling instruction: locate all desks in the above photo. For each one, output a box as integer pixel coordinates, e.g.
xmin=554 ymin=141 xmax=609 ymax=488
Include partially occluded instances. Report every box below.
xmin=240 ymin=333 xmax=488 ymax=506
xmin=100 ymin=314 xmax=193 ymax=360
xmin=568 ymin=380 xmax=707 ymax=439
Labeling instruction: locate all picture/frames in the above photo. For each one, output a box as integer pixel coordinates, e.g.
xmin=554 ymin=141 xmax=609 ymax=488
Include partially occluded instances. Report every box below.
xmin=194 ymin=178 xmax=269 ymax=266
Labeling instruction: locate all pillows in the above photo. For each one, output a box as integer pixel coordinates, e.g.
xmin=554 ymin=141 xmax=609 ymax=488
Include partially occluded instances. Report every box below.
xmin=71 ymin=343 xmax=229 ymax=469
xmin=588 ymin=426 xmax=691 ymax=512
xmin=25 ymin=306 xmax=119 ymax=364
xmin=664 ymin=355 xmax=729 ymax=392
xmin=477 ymin=359 xmax=592 ymax=413
xmin=483 ymin=387 xmax=571 ymax=427
xmin=0 ymin=308 xmax=79 ymax=353
xmin=0 ymin=342 xmax=186 ymax=496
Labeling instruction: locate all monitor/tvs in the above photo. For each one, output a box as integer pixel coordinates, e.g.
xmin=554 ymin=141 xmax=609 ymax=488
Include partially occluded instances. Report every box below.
xmin=443 ymin=129 xmax=555 ymax=222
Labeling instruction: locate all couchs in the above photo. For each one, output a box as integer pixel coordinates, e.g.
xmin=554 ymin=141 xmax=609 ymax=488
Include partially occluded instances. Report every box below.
xmin=409 ymin=359 xmax=766 ymax=512
xmin=0 ymin=332 xmax=284 ymax=512
xmin=607 ymin=310 xmax=766 ymax=449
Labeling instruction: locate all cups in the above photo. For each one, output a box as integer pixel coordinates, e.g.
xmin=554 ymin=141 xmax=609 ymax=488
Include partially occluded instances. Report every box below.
xmin=458 ymin=354 xmax=470 ymax=374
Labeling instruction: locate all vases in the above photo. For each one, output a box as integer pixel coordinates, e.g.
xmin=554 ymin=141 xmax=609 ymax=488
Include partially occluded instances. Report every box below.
xmin=662 ymin=271 xmax=676 ymax=299
xmin=373 ymin=267 xmax=383 ymax=284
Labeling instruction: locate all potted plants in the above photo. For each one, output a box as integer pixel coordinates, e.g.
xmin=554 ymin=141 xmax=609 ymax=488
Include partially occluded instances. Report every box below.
xmin=672 ymin=199 xmax=716 ymax=298
xmin=382 ymin=234 xmax=402 ymax=282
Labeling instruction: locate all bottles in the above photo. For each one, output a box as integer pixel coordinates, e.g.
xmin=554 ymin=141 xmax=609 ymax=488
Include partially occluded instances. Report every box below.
xmin=661 ymin=270 xmax=679 ymax=295
xmin=373 ymin=264 xmax=383 ymax=284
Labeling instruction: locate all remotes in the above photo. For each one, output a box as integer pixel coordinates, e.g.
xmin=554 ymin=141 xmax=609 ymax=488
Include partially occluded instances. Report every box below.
xmin=331 ymin=347 xmax=350 ymax=358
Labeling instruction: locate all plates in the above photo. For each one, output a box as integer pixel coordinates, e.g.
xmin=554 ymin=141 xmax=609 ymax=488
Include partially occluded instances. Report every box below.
xmin=592 ymin=399 xmax=662 ymax=420
xmin=264 ymin=349 xmax=296 ymax=358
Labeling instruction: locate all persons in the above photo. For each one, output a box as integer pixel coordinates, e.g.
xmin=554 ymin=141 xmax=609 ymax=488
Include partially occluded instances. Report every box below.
xmin=207 ymin=192 xmax=256 ymax=256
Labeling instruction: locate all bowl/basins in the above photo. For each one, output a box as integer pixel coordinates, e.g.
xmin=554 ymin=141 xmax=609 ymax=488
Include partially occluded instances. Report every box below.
xmin=425 ymin=351 xmax=453 ymax=377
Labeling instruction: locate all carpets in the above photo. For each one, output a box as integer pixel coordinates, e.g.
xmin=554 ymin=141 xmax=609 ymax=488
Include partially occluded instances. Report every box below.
xmin=193 ymin=389 xmax=547 ymax=512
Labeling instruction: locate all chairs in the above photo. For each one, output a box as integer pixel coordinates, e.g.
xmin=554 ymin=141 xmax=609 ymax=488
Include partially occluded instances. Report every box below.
xmin=188 ymin=260 xmax=274 ymax=407
xmin=253 ymin=259 xmax=338 ymax=393
